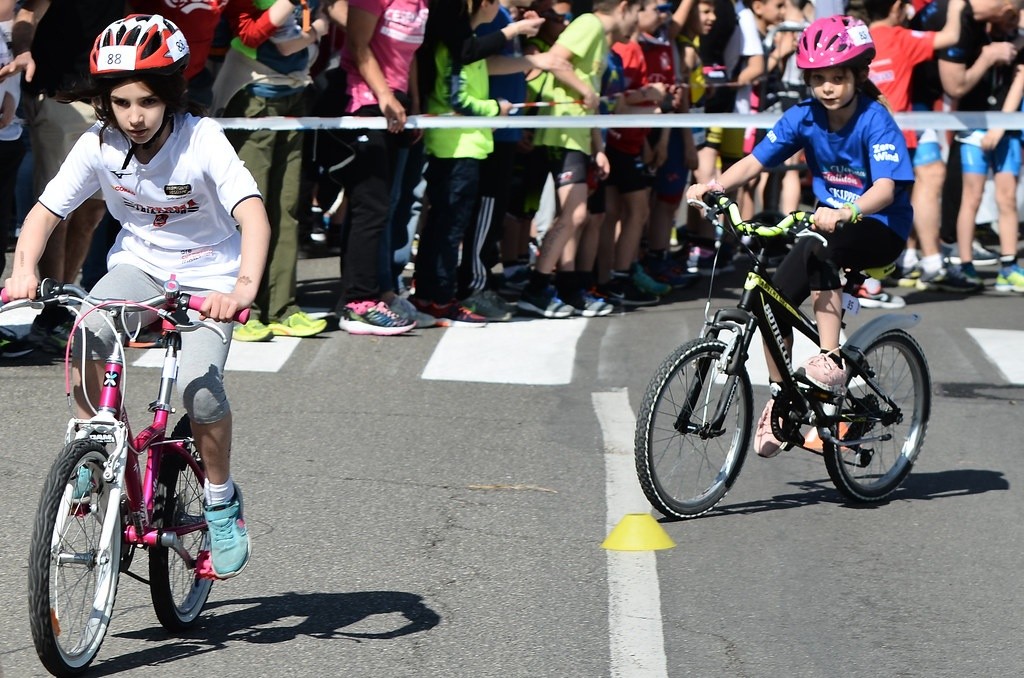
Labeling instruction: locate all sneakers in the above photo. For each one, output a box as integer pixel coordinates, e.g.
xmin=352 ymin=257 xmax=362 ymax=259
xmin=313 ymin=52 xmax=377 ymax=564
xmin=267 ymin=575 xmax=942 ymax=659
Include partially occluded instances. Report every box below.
xmin=70 ymin=465 xmax=91 ymax=504
xmin=754 ymin=397 xmax=789 ymax=459
xmin=794 ymin=348 xmax=846 ymax=395
xmin=29 ymin=316 xmax=75 ymax=355
xmin=858 ymin=260 xmax=1023 ymax=308
xmin=231 ymin=235 xmax=738 ymax=344
xmin=203 ymin=483 xmax=252 ymax=580
xmin=0 ymin=328 xmax=34 ymax=357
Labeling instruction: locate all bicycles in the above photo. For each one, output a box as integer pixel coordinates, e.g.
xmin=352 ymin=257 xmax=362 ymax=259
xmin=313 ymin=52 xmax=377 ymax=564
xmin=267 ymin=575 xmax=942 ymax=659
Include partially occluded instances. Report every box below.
xmin=0 ymin=279 xmax=249 ymax=678
xmin=634 ymin=191 xmax=932 ymax=520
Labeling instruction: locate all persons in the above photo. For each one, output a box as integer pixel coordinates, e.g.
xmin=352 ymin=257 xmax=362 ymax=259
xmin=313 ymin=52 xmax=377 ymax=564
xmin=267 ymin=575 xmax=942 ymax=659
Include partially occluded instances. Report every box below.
xmin=687 ymin=15 xmax=915 ymax=458
xmin=5 ymin=14 xmax=271 ymax=578
xmin=0 ymin=0 xmax=1024 ymax=357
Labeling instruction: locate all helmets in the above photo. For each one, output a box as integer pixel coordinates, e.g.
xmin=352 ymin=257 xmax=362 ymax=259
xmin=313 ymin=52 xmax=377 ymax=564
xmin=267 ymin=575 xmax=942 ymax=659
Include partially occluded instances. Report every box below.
xmin=90 ymin=13 xmax=189 ymax=74
xmin=797 ymin=16 xmax=877 ymax=71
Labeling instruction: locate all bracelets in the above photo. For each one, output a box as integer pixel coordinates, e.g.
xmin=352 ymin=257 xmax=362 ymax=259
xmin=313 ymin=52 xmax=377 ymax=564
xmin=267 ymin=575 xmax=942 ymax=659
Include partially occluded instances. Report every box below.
xmin=842 ymin=202 xmax=863 ymax=223
